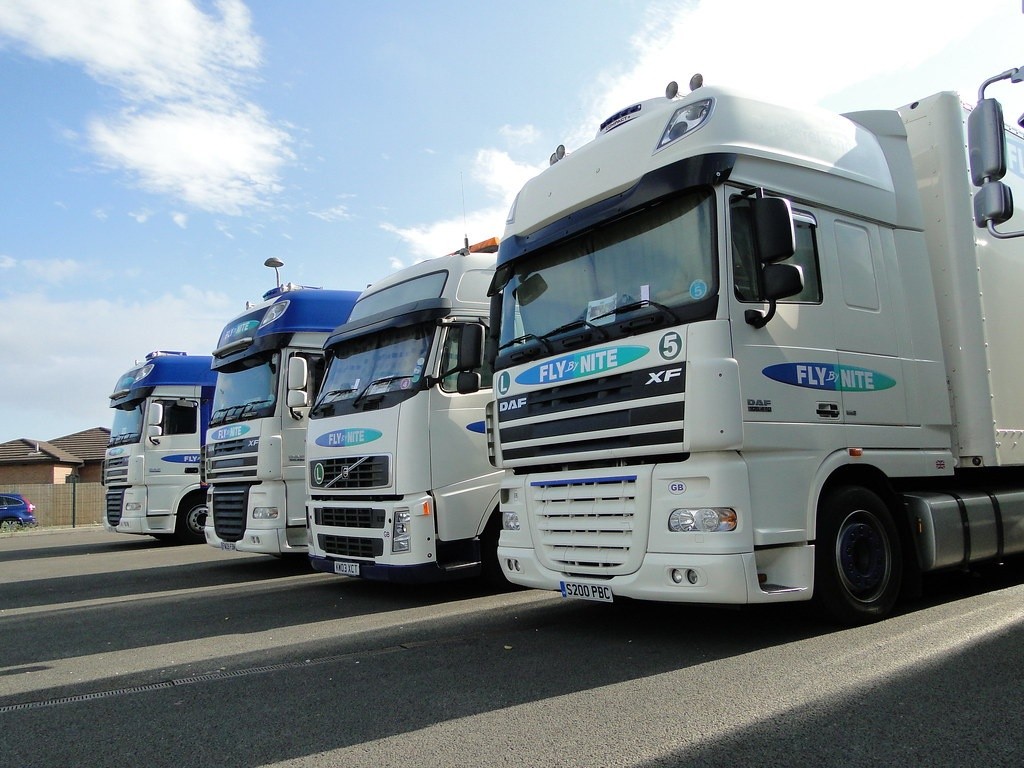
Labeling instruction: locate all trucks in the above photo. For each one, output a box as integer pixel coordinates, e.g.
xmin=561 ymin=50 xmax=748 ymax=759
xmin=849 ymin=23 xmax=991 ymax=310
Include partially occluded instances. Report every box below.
xmin=196 ymin=255 xmax=365 ymax=578
xmin=303 ymin=235 xmax=525 ymax=590
xmin=480 ymin=65 xmax=1024 ymax=629
xmin=101 ymin=351 xmax=220 ymax=545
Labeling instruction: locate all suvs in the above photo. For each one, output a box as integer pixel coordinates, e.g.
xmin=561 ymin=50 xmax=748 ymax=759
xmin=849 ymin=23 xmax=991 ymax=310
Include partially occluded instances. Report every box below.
xmin=1 ymin=493 xmax=37 ymax=530
